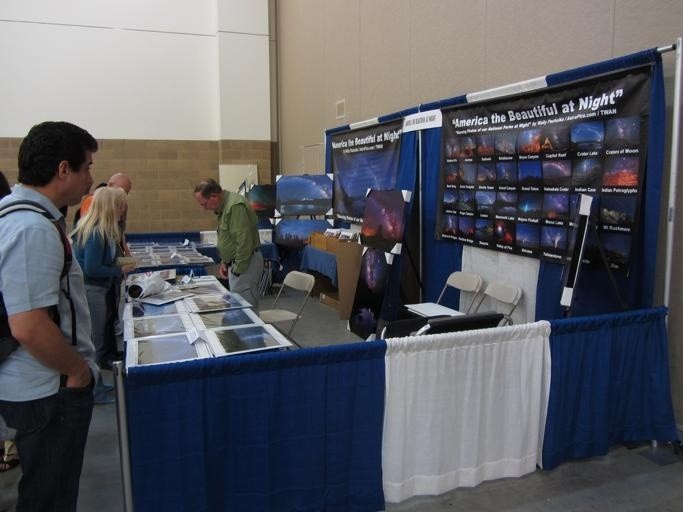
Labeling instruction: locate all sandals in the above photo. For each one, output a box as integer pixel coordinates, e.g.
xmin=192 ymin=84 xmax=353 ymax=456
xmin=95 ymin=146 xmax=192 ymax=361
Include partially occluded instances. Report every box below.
xmin=0 ymin=457 xmax=19 ymax=471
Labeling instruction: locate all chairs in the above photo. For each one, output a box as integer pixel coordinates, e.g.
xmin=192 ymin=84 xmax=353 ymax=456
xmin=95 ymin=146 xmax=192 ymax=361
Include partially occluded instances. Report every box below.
xmin=260 ymin=270 xmax=316 ymax=349
xmin=472 ymin=282 xmax=524 ymax=328
xmin=378 ymin=313 xmax=512 ymax=341
xmin=402 ymin=270 xmax=481 ymax=319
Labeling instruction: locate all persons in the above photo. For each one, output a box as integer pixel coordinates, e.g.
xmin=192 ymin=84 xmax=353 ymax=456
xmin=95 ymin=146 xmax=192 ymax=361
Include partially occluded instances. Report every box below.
xmin=193 ymin=178 xmax=265 ymax=320
xmin=67 ymin=186 xmax=137 ymax=368
xmin=72 ymin=183 xmax=106 ymax=233
xmin=0 ymin=120 xmax=100 ymax=512
xmin=1 ymin=440 xmax=20 ymax=473
xmin=106 ymin=172 xmax=132 ymax=258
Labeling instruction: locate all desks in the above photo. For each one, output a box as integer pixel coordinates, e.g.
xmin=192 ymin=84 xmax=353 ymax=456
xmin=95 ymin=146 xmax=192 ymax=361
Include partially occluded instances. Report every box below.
xmin=118 ymin=237 xmax=277 ymax=295
xmin=123 ymin=274 xmax=298 ymax=380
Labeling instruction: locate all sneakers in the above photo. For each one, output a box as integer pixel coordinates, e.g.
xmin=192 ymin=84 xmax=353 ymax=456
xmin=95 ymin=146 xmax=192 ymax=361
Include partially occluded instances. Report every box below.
xmin=102 ymin=383 xmax=116 ymax=403
